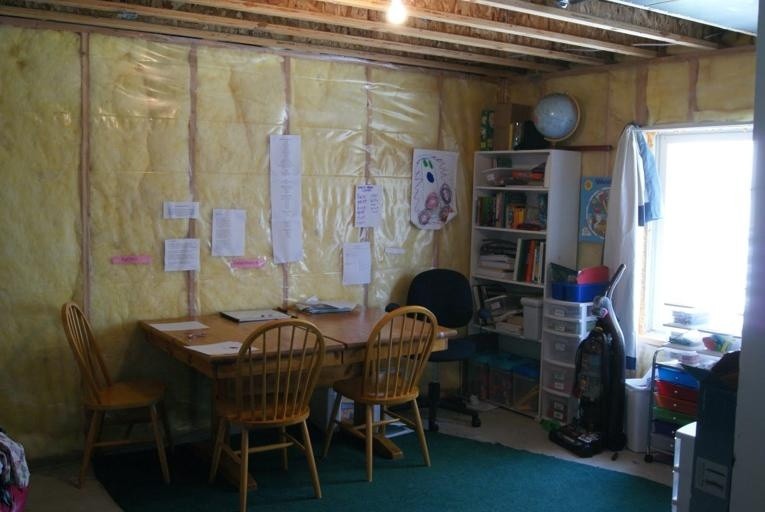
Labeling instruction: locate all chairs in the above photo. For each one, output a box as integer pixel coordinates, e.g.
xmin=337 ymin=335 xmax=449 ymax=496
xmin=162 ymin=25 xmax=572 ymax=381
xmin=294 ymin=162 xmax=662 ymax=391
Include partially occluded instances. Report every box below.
xmin=209 ymin=319 xmax=325 ymax=512
xmin=322 ymin=305 xmax=437 ymax=482
xmin=61 ymin=301 xmax=179 ymax=490
xmin=385 ymin=268 xmax=499 ymax=431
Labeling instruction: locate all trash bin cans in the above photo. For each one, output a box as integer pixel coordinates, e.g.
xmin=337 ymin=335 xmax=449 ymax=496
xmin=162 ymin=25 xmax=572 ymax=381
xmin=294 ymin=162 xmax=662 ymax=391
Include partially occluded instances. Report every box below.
xmin=625 ymin=378 xmax=657 ymax=452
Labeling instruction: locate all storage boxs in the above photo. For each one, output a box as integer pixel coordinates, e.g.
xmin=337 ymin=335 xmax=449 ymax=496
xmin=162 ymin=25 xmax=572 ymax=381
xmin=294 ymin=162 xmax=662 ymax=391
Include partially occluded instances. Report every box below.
xmin=541 ymin=298 xmax=610 ymax=427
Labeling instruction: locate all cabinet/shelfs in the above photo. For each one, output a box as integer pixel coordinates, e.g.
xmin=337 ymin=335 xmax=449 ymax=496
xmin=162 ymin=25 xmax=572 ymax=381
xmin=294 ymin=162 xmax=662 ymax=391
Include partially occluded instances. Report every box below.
xmin=644 ymin=302 xmax=742 ymax=464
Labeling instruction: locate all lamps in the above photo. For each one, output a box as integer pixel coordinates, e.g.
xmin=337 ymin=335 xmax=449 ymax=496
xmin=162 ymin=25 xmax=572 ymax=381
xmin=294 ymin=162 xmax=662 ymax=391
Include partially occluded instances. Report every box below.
xmin=467 ymin=149 xmax=582 ymax=423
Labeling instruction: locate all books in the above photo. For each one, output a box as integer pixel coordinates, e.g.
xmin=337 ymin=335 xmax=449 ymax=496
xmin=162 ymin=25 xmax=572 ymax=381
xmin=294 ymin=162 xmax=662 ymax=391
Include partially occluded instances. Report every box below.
xmin=469 ymin=151 xmax=548 ymax=342
xmin=479 ymin=108 xmax=492 ymax=150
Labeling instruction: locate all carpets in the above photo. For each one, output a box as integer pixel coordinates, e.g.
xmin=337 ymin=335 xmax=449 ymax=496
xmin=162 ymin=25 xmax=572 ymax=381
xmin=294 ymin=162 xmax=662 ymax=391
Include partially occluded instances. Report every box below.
xmin=92 ymin=414 xmax=725 ymax=512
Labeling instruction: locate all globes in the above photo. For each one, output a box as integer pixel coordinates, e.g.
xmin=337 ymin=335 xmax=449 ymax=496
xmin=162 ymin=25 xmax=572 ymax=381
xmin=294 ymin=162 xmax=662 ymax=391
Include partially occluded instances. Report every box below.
xmin=532 ymin=91 xmax=580 ymax=149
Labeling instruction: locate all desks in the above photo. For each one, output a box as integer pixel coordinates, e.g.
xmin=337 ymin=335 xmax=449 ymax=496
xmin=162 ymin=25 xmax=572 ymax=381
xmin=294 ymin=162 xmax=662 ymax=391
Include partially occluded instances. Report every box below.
xmin=137 ymin=302 xmax=457 ymax=493
